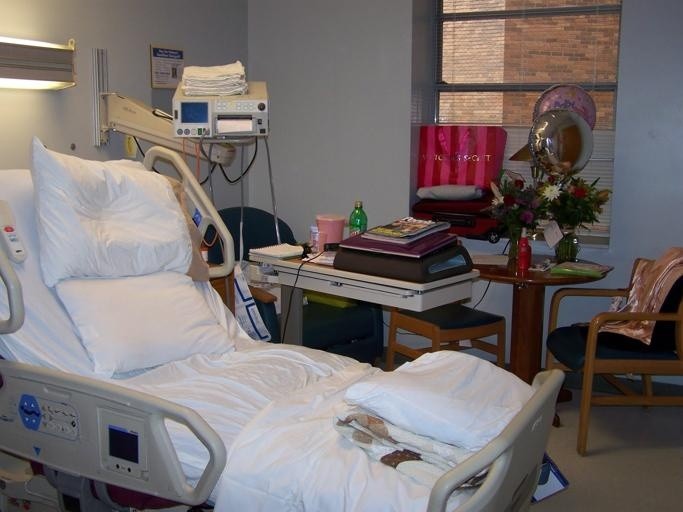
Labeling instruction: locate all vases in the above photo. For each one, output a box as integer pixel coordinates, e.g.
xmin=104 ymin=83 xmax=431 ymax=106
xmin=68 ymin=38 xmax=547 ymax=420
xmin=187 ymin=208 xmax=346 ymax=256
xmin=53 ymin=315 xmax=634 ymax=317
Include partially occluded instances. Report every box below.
xmin=505 ymin=222 xmax=535 ymax=277
xmin=555 ymin=225 xmax=582 ymax=263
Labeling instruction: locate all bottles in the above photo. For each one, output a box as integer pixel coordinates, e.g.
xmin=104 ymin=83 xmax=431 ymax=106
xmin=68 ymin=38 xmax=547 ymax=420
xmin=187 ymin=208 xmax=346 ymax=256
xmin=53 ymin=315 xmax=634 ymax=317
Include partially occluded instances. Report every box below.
xmin=518 ymin=227 xmax=529 ymax=270
xmin=200 ymin=241 xmax=208 ymax=265
xmin=349 ymin=200 xmax=367 ymax=238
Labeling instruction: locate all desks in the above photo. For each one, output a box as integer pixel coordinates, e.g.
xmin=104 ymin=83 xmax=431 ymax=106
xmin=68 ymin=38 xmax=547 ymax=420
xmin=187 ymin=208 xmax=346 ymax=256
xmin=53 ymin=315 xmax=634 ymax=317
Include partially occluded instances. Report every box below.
xmin=450 ymin=254 xmax=621 ymax=427
xmin=249 ymin=240 xmax=480 ymax=346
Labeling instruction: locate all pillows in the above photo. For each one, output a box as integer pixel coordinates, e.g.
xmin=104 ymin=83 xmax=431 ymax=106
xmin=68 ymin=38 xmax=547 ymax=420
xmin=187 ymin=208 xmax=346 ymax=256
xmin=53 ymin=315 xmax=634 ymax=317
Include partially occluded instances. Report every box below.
xmin=25 ymin=133 xmax=212 ymax=290
xmin=47 ymin=270 xmax=243 ymax=378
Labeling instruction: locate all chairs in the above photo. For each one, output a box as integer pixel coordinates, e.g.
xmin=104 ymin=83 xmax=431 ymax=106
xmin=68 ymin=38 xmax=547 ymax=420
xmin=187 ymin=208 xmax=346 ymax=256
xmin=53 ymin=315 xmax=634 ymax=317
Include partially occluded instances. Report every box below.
xmin=544 ymin=246 xmax=683 ymax=456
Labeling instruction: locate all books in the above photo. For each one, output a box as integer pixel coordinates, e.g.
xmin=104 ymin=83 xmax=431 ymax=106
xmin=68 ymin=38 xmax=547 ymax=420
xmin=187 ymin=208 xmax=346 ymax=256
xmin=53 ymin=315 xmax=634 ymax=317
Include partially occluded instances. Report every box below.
xmin=360 ymin=215 xmax=451 ymax=245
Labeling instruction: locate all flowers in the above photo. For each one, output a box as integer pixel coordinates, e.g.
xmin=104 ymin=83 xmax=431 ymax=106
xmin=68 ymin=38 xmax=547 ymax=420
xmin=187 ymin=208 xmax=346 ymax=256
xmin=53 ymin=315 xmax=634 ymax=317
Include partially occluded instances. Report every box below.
xmin=533 ymin=171 xmax=615 ymax=233
xmin=460 ymin=168 xmax=543 ymax=240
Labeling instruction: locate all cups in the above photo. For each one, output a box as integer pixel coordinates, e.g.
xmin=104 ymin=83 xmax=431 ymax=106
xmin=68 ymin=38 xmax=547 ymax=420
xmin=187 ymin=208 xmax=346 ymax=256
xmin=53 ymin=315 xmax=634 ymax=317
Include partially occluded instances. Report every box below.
xmin=316 ymin=214 xmax=346 ymax=257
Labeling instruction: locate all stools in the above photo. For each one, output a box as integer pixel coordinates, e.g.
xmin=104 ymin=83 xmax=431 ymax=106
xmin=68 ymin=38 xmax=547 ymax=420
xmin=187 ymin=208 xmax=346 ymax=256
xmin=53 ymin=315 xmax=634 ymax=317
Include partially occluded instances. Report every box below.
xmin=385 ymin=302 xmax=507 ymax=372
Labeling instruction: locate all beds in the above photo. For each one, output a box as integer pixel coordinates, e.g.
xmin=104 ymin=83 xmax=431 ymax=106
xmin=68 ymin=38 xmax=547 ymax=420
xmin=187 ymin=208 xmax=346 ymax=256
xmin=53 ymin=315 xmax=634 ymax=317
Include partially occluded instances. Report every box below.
xmin=0 ymin=143 xmax=567 ymax=512
